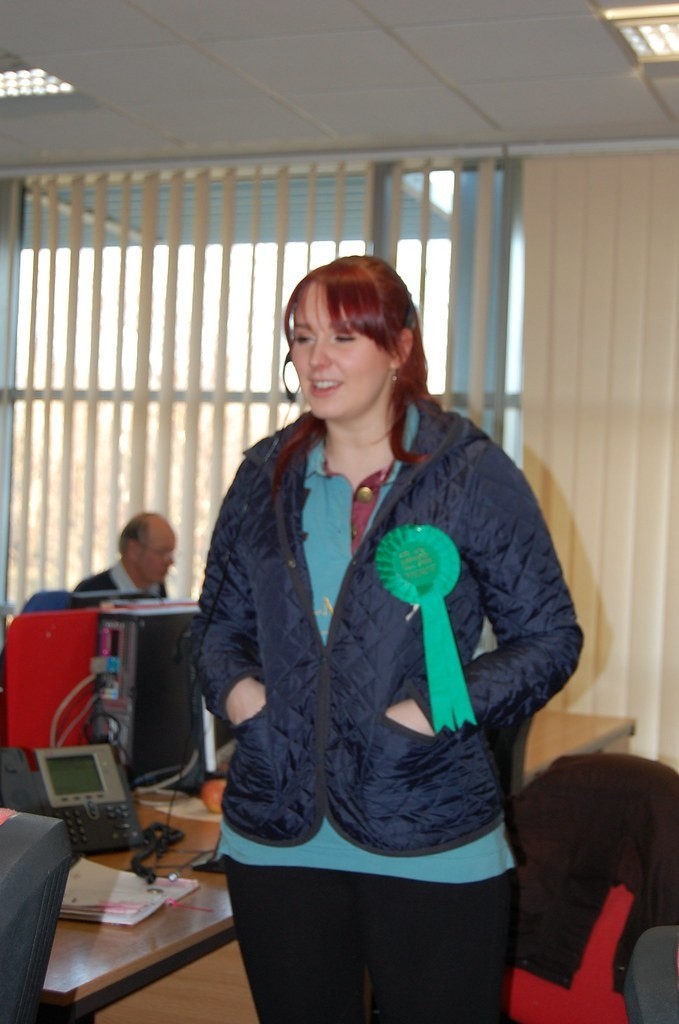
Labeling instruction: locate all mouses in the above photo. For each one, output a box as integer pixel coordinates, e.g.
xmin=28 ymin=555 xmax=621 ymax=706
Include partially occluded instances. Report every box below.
xmin=190 ymin=851 xmax=226 ymax=873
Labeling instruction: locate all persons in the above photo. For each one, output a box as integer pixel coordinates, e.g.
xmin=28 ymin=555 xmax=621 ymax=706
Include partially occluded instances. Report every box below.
xmin=190 ymin=255 xmax=585 ymax=1024
xmin=72 ymin=513 xmax=176 ymax=599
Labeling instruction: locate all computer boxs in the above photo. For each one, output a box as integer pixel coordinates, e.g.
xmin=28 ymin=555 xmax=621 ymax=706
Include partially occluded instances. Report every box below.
xmin=88 ymin=605 xmax=209 ymax=790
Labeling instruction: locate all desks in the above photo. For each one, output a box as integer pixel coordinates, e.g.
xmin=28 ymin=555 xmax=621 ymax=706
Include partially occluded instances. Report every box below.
xmin=41 ymin=704 xmax=639 ymax=1024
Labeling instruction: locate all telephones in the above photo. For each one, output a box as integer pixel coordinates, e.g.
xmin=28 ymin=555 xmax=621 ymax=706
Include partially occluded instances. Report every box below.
xmin=0 ymin=743 xmax=146 ymax=857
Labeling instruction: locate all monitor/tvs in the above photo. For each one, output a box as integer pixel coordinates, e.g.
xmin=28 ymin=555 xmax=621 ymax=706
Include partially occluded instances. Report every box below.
xmin=7 ymin=601 xmax=207 ymax=772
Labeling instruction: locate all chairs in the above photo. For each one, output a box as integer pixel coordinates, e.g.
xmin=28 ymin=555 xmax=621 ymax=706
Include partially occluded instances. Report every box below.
xmin=501 ymin=750 xmax=678 ymax=1024
